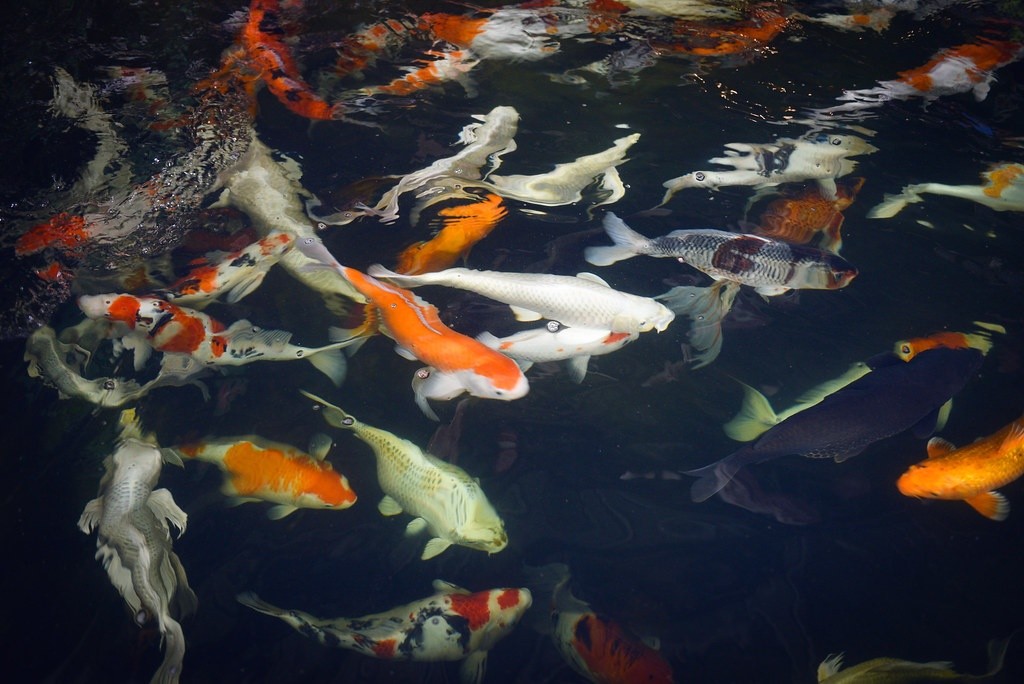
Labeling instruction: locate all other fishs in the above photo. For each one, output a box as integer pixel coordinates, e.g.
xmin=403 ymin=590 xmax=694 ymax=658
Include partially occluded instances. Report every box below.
xmin=0 ymin=0 xmax=1024 ymax=682
